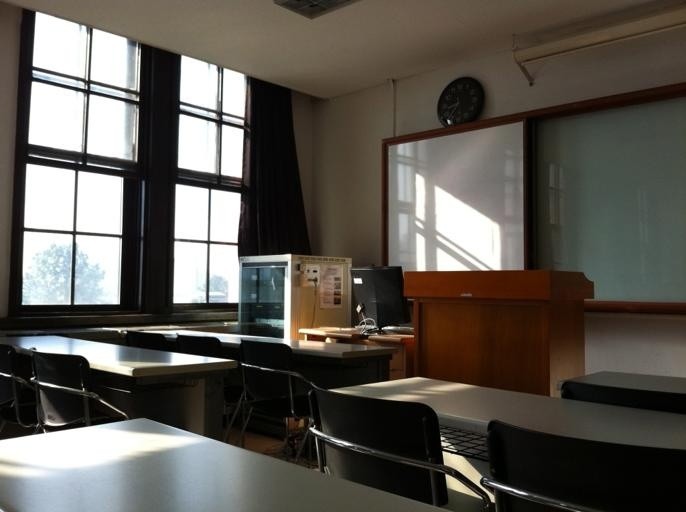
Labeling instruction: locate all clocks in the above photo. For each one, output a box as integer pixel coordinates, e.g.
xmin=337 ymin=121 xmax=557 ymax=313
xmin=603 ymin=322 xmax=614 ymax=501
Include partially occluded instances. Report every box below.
xmin=437 ymin=77 xmax=482 ymax=127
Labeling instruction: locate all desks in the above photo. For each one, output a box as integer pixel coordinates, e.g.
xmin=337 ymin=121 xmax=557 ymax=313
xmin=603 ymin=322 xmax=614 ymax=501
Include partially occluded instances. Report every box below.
xmin=2 ymin=418 xmax=448 ymax=510
xmin=327 ymin=376 xmax=686 ymax=458
xmin=558 ymin=371 xmax=685 ymax=409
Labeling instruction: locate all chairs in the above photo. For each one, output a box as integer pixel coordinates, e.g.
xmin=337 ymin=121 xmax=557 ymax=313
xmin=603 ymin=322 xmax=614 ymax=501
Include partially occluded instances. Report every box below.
xmin=481 ymin=417 xmax=685 ymax=510
xmin=310 ymin=392 xmax=491 ymax=510
xmin=0 ymin=329 xmax=398 ymax=444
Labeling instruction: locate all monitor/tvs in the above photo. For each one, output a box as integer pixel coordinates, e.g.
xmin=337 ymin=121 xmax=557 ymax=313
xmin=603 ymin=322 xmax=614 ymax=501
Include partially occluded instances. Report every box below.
xmin=350 ymin=266 xmax=412 ymax=333
